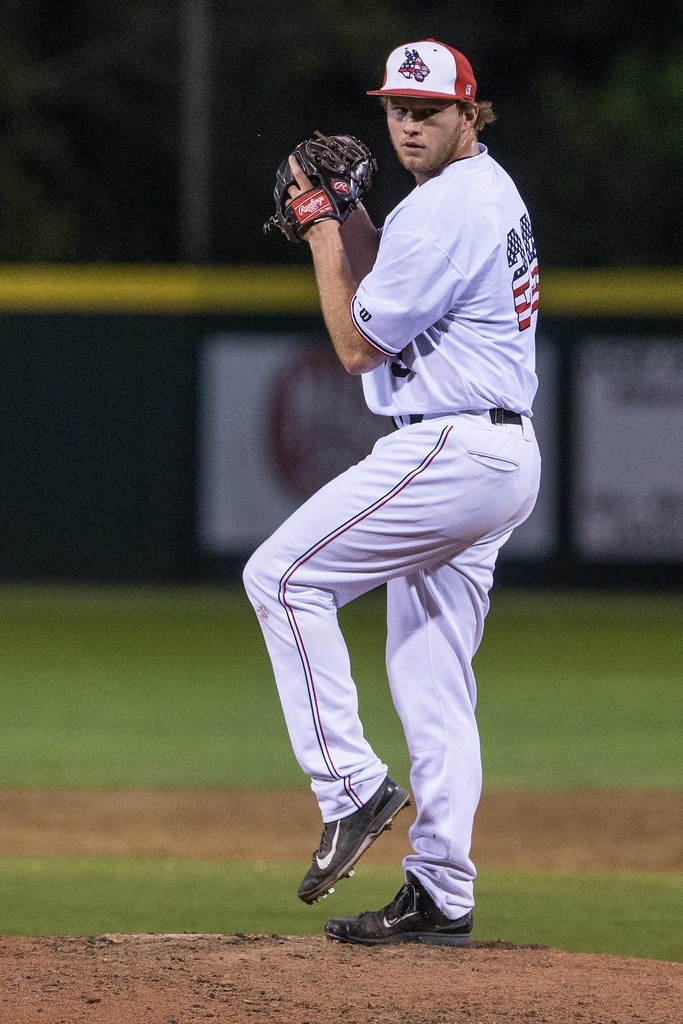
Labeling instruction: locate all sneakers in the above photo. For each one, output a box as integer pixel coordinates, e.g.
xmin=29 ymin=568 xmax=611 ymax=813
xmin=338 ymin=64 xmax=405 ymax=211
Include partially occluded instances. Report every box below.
xmin=297 ymin=772 xmax=412 ymax=905
xmin=323 ymin=870 xmax=473 ymax=948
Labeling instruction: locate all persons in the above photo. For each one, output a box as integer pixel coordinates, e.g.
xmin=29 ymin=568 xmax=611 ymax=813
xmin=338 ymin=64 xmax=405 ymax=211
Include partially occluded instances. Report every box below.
xmin=243 ymin=38 xmax=542 ymax=945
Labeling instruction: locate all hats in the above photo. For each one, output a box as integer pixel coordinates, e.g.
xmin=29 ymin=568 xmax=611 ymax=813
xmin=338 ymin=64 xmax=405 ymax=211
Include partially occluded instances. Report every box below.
xmin=366 ymin=38 xmax=477 ymax=102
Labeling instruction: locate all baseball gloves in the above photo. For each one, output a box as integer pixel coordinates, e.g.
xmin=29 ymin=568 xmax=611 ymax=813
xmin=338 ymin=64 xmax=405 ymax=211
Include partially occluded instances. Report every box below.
xmin=274 ymin=135 xmax=378 ymax=243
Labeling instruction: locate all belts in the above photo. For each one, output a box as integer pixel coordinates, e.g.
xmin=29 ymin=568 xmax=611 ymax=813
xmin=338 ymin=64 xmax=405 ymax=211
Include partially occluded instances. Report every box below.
xmin=390 ymin=407 xmax=522 ymax=430
